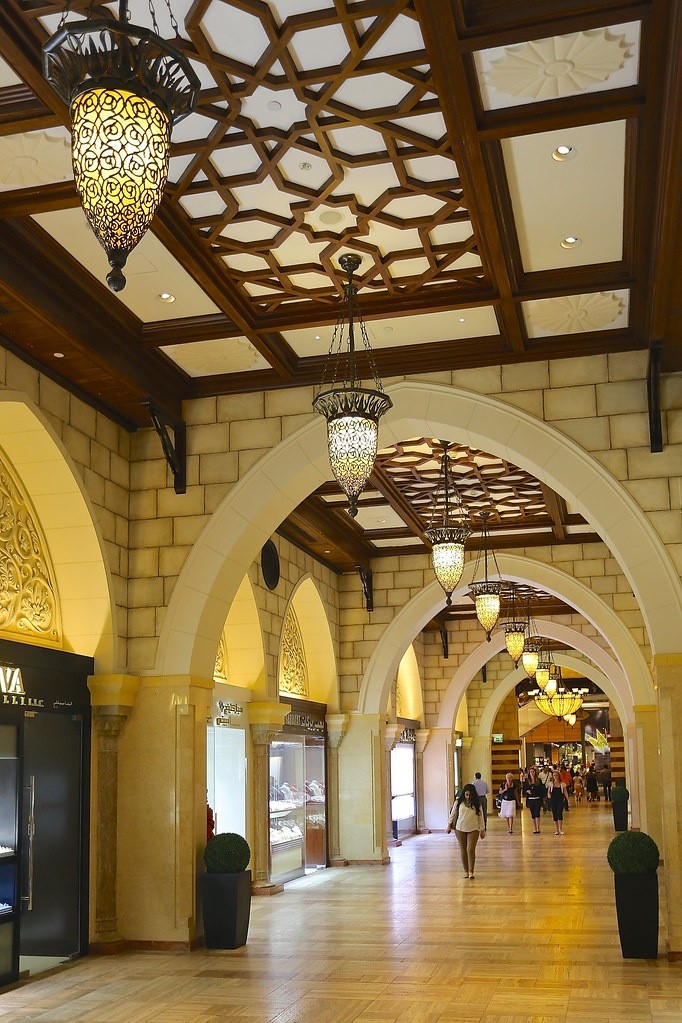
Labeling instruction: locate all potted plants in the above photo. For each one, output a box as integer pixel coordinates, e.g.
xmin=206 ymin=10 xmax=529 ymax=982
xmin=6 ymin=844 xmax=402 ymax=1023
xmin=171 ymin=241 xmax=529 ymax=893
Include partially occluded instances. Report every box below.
xmin=608 ymin=832 xmax=661 ymax=960
xmin=611 ymin=777 xmax=629 ymax=832
xmin=202 ymin=832 xmax=252 ymax=951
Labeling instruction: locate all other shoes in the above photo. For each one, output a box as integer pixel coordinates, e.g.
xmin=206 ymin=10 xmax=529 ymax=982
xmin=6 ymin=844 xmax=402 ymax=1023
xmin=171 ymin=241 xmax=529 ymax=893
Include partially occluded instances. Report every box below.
xmin=463 ymin=873 xmax=469 ymax=878
xmin=543 ymin=809 xmax=551 ymax=814
xmin=509 ymin=829 xmax=513 ymax=834
xmin=561 ymin=831 xmax=564 ymax=835
xmin=469 ymin=873 xmax=475 ymax=880
xmin=589 ymin=799 xmax=595 ymax=802
xmin=577 ymin=797 xmax=581 ymax=802
xmin=555 ymin=832 xmax=559 ymax=835
xmin=565 ymin=808 xmax=570 ymax=811
xmin=533 ymin=831 xmax=540 ymax=834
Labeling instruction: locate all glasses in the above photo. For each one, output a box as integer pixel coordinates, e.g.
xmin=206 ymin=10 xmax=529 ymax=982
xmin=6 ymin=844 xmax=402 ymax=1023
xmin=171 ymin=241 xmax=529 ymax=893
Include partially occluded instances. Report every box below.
xmin=465 ymin=793 xmax=472 ymax=796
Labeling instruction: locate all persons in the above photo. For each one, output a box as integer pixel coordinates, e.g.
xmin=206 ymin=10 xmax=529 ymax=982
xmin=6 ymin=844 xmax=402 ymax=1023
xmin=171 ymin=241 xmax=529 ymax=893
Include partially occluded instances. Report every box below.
xmin=270 ymin=779 xmax=325 ymax=843
xmin=206 ymin=789 xmax=214 ymax=842
xmin=446 ymin=784 xmax=485 ymax=879
xmin=473 ymin=760 xmax=611 ymax=835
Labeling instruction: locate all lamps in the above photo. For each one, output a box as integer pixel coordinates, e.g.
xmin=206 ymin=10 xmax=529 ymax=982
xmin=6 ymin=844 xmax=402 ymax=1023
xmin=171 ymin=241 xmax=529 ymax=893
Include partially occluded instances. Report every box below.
xmin=423 ymin=442 xmax=479 ymax=610
xmin=526 ymin=637 xmax=590 ymax=730
xmin=468 ymin=512 xmax=507 ymax=644
xmin=498 ymin=588 xmax=530 ymax=670
xmin=313 ymin=253 xmax=394 ymax=519
xmin=41 ymin=0 xmax=202 ymax=292
xmin=521 ymin=601 xmax=538 ymax=684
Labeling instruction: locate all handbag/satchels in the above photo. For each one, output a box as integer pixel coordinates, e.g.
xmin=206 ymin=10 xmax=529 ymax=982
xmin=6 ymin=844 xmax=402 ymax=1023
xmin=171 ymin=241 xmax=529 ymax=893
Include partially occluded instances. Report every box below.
xmin=496 ymin=794 xmax=502 ymax=808
xmin=542 ymin=783 xmax=546 ymax=798
xmin=451 ymin=816 xmax=457 ymax=830
